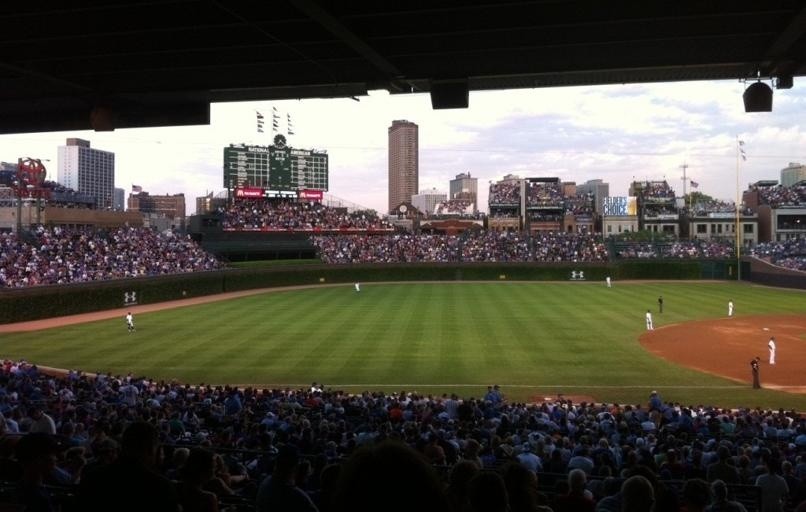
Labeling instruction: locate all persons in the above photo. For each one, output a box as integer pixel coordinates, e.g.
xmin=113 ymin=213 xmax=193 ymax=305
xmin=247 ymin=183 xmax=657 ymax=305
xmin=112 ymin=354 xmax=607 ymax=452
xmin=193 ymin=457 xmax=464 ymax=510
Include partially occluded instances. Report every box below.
xmin=750 ymin=182 xmax=805 ymax=209
xmin=728 ymin=299 xmax=734 ymax=316
xmin=309 ymin=226 xmax=610 ymax=265
xmin=746 ymin=236 xmax=806 ymax=271
xmin=619 ymin=238 xmax=735 ymax=259
xmin=525 ymin=182 xmax=595 ymax=225
xmin=0 ymin=356 xmax=806 ymax=511
xmin=633 ymin=182 xmax=676 ymax=216
xmin=1 ymin=182 xmax=124 ymax=213
xmin=125 ymin=311 xmax=137 ymax=335
xmin=768 ymin=336 xmax=775 ymax=365
xmin=490 ymin=183 xmax=522 ymax=219
xmin=646 ymin=309 xmax=654 ymax=331
xmin=425 ymin=198 xmax=473 ymax=218
xmin=680 ymin=198 xmax=752 ymax=219
xmin=1 ymin=221 xmax=231 ymax=288
xmin=217 ymin=195 xmax=407 ymax=230
xmin=658 ymin=296 xmax=663 ymax=313
